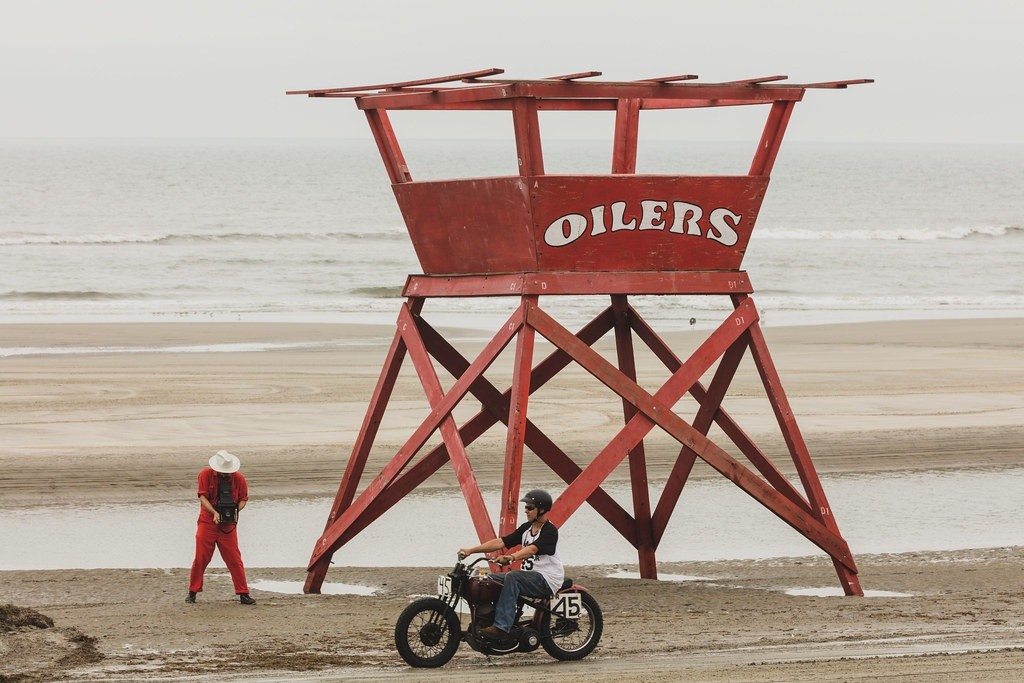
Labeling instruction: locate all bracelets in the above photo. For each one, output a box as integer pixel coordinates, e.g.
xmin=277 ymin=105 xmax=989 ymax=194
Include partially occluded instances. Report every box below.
xmin=510 ymin=554 xmax=516 ymax=562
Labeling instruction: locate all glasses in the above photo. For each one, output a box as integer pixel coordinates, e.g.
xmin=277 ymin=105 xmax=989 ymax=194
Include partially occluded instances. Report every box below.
xmin=525 ymin=505 xmax=538 ymax=511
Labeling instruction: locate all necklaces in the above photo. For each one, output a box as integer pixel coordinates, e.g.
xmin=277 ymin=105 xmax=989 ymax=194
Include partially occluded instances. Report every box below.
xmin=532 ymin=522 xmax=542 ymax=533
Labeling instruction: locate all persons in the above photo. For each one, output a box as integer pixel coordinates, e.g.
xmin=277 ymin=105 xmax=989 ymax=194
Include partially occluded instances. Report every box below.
xmin=456 ymin=489 xmax=565 ymax=640
xmin=184 ymin=449 xmax=258 ymax=605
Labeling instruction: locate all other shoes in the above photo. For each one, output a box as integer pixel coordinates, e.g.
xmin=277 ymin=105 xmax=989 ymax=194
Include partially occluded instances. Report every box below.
xmin=240 ymin=593 xmax=256 ymax=604
xmin=185 ymin=591 xmax=196 ymax=603
xmin=479 ymin=626 xmax=507 ymax=640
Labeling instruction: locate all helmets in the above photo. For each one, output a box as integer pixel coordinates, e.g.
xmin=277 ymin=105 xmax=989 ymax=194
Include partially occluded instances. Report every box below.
xmin=520 ymin=489 xmax=552 ymax=511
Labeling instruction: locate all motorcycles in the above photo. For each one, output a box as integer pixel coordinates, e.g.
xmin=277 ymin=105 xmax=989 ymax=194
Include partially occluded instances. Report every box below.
xmin=394 ymin=552 xmax=604 ymax=670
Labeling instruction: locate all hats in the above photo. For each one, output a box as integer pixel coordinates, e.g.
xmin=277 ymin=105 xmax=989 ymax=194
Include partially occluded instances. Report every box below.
xmin=209 ymin=451 xmax=240 ymax=473
